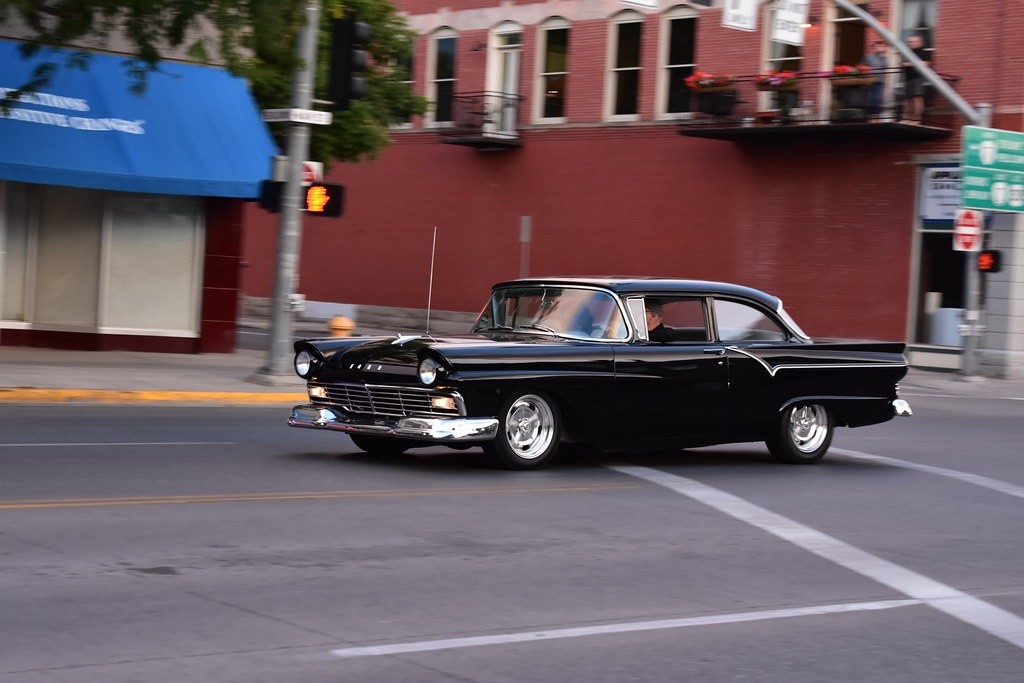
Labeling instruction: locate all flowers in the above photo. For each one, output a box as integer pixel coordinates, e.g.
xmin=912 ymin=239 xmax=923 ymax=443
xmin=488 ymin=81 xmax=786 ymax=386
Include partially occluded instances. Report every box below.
xmin=756 ymin=72 xmax=799 ymax=85
xmin=818 ymin=63 xmax=873 ymax=77
xmin=684 ymin=72 xmax=734 ymax=89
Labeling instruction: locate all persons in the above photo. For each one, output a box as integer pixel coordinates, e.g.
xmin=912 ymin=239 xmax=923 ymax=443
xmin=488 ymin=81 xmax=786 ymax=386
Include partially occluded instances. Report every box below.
xmin=602 ymin=302 xmax=675 ymax=342
xmin=860 ymin=37 xmax=931 ymax=125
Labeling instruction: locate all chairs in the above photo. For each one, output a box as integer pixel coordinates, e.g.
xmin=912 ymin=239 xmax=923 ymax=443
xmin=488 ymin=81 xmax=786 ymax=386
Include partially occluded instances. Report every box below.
xmin=570 ymin=305 xmax=593 ymax=333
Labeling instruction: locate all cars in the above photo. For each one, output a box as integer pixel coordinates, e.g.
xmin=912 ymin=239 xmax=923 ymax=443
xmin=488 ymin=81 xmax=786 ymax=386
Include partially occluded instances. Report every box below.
xmin=284 ymin=275 xmax=913 ymax=472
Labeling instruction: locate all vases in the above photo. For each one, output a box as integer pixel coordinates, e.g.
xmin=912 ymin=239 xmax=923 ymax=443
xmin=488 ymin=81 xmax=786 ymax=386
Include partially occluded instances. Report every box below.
xmin=759 ymin=85 xmax=799 ymax=108
xmin=698 ymin=89 xmax=736 ymax=115
xmin=831 ymin=78 xmax=884 ymax=112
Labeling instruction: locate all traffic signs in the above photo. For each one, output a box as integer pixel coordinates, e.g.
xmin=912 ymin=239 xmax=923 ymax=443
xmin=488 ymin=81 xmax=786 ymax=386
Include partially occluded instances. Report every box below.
xmin=958 ymin=121 xmax=1024 ymax=213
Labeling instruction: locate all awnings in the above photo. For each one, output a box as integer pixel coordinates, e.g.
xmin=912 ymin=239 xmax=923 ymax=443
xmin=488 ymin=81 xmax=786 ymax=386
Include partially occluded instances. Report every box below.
xmin=0 ymin=36 xmax=285 ymax=201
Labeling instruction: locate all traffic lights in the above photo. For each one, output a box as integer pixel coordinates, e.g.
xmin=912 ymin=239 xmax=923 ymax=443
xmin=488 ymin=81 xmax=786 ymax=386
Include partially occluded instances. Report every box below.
xmin=303 ymin=182 xmax=347 ymax=217
xmin=977 ymin=250 xmax=1000 ymax=274
xmin=327 ymin=10 xmax=375 ymax=116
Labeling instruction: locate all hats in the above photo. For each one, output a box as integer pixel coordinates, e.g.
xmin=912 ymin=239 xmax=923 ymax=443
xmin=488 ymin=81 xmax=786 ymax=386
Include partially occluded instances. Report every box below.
xmin=645 ymin=298 xmax=663 ymax=317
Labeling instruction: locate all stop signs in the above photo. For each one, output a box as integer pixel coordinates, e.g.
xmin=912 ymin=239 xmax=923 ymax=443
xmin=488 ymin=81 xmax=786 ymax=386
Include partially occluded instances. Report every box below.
xmin=950 ymin=208 xmax=984 ymax=250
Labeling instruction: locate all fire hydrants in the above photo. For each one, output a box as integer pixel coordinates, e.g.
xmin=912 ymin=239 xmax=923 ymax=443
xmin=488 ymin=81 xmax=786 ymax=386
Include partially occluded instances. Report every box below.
xmin=328 ymin=315 xmax=359 ymax=340
